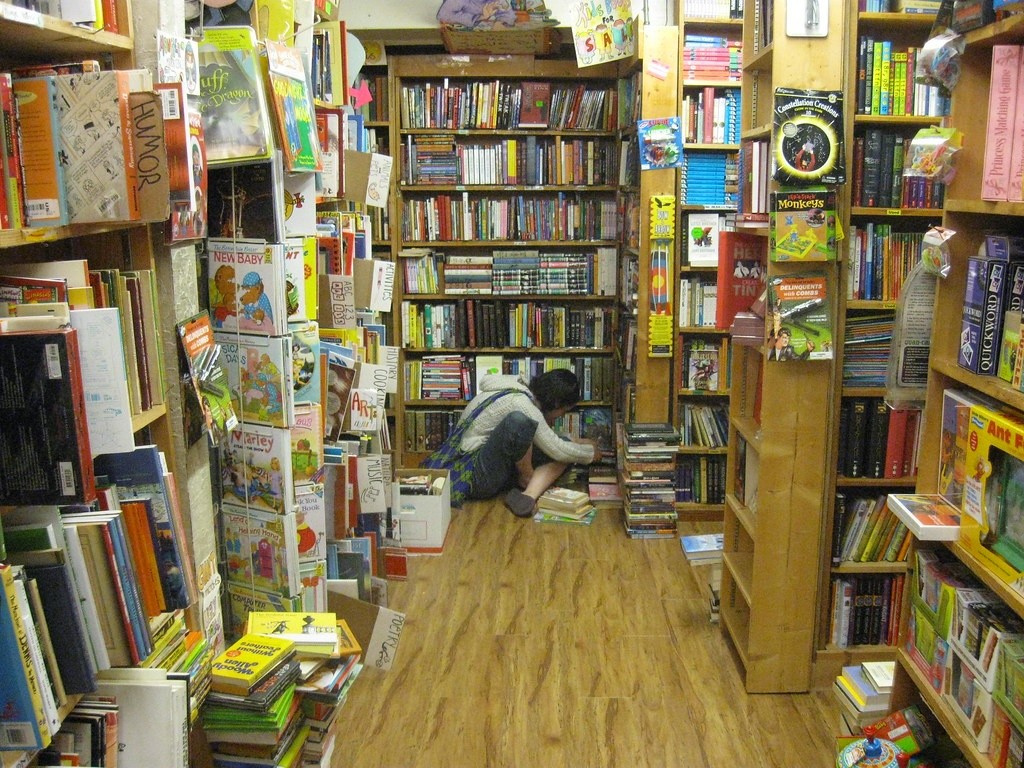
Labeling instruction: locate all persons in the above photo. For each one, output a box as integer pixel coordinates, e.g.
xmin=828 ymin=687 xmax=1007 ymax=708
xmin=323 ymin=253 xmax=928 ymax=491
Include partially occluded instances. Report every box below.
xmin=455 ymin=368 xmax=602 ymax=516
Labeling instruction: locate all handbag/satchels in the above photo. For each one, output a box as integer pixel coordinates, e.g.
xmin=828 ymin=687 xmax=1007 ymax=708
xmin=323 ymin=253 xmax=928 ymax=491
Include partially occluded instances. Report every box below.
xmin=417 ymin=432 xmax=479 ymax=509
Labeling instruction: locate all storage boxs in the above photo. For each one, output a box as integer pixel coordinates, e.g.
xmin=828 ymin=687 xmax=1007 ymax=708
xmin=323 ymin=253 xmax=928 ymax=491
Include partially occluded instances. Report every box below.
xmin=890 ymin=42 xmax=1024 ymax=768
xmin=439 ymin=24 xmax=562 ymax=54
xmin=392 ymin=468 xmax=452 ymax=557
xmin=769 ymin=185 xmax=837 ymax=263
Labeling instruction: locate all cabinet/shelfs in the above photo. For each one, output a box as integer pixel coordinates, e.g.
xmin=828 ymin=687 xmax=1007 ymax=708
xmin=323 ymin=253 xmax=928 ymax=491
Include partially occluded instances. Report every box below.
xmin=908 ymin=11 xmax=1024 ymax=768
xmin=812 ymin=0 xmax=951 ymax=695
xmin=0 ymin=0 xmax=202 ymax=768
xmin=358 ymin=55 xmax=400 ymax=471
xmin=395 ymin=54 xmax=620 ymax=469
xmin=624 ymin=0 xmax=677 ymax=424
xmin=718 ymin=0 xmax=846 ymax=698
xmin=672 ymin=0 xmax=743 ymax=513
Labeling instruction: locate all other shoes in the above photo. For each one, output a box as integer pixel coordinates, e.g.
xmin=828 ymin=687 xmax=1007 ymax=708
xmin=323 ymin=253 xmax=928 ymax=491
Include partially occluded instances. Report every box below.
xmin=505 ymin=489 xmax=536 ymax=517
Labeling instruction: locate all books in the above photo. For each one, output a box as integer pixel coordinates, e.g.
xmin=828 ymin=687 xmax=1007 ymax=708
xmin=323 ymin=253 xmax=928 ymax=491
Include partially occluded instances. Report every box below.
xmin=0 ymin=1 xmax=395 ymax=768
xmin=680 ymin=534 xmax=723 ymax=566
xmin=675 ymin=1 xmax=773 ymax=516
xmin=827 ymin=0 xmax=952 ymax=646
xmin=396 ymin=71 xmax=641 ymax=525
xmin=622 ymin=423 xmax=682 ymax=539
xmin=832 ymin=661 xmax=895 ymax=737
